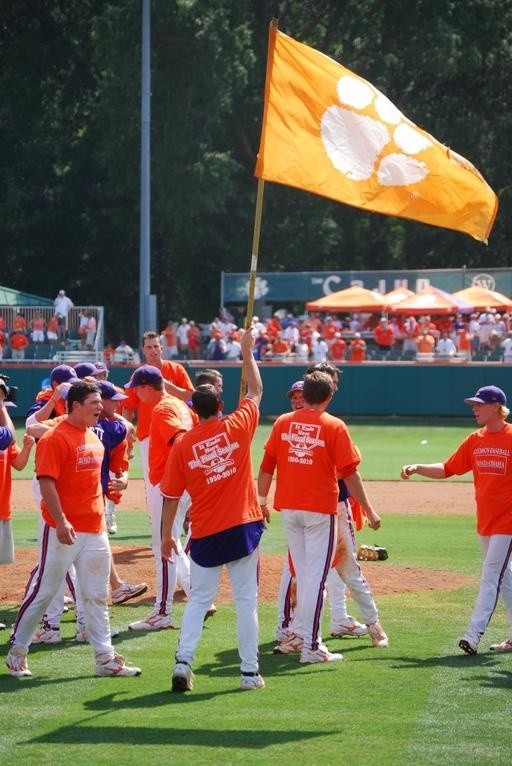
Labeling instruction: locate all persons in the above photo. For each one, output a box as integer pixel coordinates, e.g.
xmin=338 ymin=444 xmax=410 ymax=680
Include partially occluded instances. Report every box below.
xmin=1 ymin=332 xmax=391 ymax=691
xmin=398 ymin=385 xmax=512 ymax=654
xmin=0 ymin=290 xmax=511 ymax=362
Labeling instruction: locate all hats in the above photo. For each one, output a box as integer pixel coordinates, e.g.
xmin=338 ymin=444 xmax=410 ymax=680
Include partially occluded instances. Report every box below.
xmin=49 ymin=362 xmax=110 ymax=384
xmin=93 ymin=381 xmax=129 ymax=400
xmin=464 ymin=383 xmax=507 ymax=406
xmin=286 ymin=379 xmax=305 ymax=399
xmin=125 ymin=362 xmax=164 ymax=390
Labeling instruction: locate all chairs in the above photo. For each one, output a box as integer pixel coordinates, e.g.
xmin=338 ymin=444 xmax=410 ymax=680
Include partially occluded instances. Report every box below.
xmin=0 ymin=326 xmax=94 ymax=364
xmin=170 ymin=331 xmax=512 ymax=365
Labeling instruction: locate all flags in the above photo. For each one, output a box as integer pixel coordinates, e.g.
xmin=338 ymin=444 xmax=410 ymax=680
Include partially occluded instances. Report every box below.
xmin=254 ymin=22 xmax=499 ymax=245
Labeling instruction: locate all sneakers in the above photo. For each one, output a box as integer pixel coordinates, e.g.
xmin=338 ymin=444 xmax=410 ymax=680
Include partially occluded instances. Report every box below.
xmin=298 ymin=645 xmax=344 ymax=663
xmin=272 ymin=627 xmax=303 ymax=654
xmin=95 ymin=657 xmax=142 ymax=677
xmin=170 ymin=663 xmax=194 ymax=691
xmin=6 ymin=650 xmax=32 ymax=677
xmin=111 ymin=581 xmax=148 ymax=605
xmin=239 ymin=672 xmax=265 ymax=690
xmin=329 ymin=617 xmax=370 ymax=638
xmin=458 ymin=637 xmax=477 ymax=655
xmin=31 ymin=626 xmax=63 ymax=645
xmin=365 ymin=621 xmax=389 ymax=648
xmin=128 ymin=611 xmax=175 ymax=632
xmin=490 ymin=639 xmax=512 ymax=651
xmin=75 ymin=622 xmax=120 ymax=642
xmin=204 ymin=601 xmax=216 ymax=623
xmin=62 ymin=595 xmax=74 ymax=613
